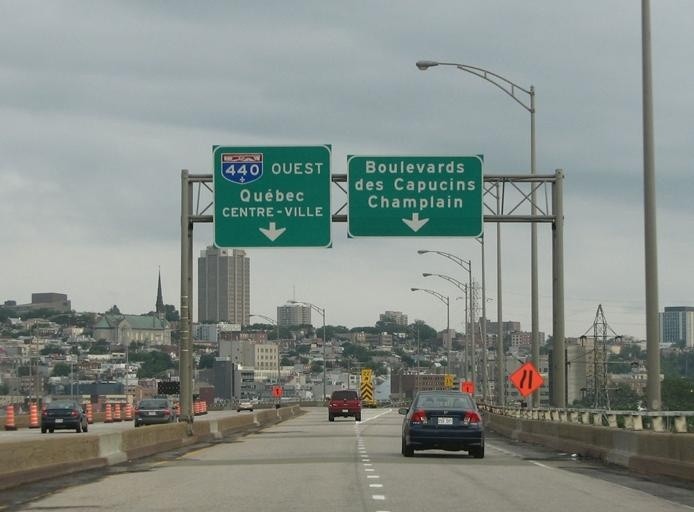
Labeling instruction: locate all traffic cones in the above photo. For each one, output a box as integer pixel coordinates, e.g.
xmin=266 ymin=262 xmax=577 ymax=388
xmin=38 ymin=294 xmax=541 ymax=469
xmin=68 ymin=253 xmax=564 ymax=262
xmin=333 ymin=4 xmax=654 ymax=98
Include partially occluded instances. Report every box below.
xmin=85 ymin=399 xmax=94 ymax=423
xmin=113 ymin=403 xmax=121 ymax=421
xmin=176 ymin=400 xmax=207 ymax=416
xmin=29 ymin=403 xmax=40 ymax=428
xmin=4 ymin=406 xmax=16 ymax=430
xmin=125 ymin=403 xmax=133 ymax=421
xmin=105 ymin=404 xmax=112 ymax=423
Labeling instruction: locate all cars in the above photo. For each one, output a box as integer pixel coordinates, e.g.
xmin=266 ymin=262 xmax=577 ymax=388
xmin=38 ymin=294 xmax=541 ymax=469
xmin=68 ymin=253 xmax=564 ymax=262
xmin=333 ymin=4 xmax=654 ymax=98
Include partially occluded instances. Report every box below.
xmin=398 ymin=390 xmax=484 ymax=458
xmin=236 ymin=398 xmax=253 ymax=412
xmin=41 ymin=400 xmax=88 ymax=432
xmin=134 ymin=398 xmax=175 ymax=427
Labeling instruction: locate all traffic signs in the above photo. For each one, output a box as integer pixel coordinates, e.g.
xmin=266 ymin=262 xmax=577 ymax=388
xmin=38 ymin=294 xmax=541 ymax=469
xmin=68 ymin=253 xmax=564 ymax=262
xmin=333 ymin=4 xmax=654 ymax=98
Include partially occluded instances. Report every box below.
xmin=211 ymin=143 xmax=331 ymax=249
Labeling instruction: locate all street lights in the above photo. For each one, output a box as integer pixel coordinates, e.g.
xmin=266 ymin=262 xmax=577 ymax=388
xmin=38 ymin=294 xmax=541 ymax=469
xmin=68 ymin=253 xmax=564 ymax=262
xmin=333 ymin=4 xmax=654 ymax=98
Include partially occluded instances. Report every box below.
xmin=286 ymin=299 xmax=325 ymax=401
xmin=346 ymin=153 xmax=484 ymax=239
xmin=411 ymin=249 xmax=477 ymax=397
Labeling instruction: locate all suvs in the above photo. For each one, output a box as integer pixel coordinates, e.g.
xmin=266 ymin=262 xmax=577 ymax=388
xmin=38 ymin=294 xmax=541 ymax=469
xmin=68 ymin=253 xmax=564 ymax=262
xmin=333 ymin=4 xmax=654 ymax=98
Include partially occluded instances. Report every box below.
xmin=325 ymin=389 xmax=361 ymax=421
